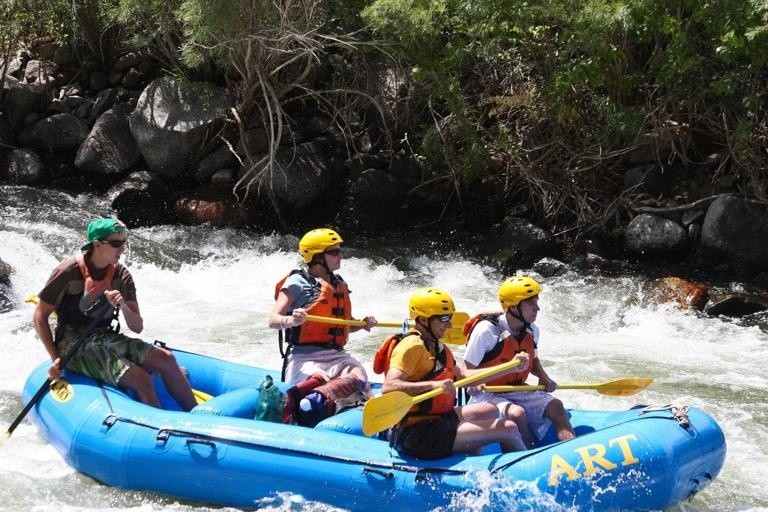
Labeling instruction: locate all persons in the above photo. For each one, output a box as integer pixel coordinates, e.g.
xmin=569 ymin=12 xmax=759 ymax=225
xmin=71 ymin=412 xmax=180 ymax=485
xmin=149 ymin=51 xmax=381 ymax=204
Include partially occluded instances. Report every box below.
xmin=34 ymin=219 xmax=198 ymax=412
xmin=269 ymin=228 xmax=379 ymax=390
xmin=382 ymin=287 xmax=527 ymax=460
xmin=461 ymin=277 xmax=575 ymax=448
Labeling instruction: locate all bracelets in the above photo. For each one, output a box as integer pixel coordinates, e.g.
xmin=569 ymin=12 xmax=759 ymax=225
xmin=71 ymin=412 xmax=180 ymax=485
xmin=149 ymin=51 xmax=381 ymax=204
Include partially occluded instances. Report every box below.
xmin=285 ymin=315 xmax=290 ymax=329
xmin=280 ymin=316 xmax=285 ymax=330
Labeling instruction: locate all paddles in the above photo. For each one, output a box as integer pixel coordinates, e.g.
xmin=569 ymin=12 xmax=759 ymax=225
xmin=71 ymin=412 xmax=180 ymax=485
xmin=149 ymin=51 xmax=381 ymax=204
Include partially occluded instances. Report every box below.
xmin=482 ymin=377 xmax=653 ymax=396
xmin=24 ymin=294 xmax=213 ymax=405
xmin=362 ymin=350 xmax=526 ymax=436
xmin=303 ymin=311 xmax=470 ymax=345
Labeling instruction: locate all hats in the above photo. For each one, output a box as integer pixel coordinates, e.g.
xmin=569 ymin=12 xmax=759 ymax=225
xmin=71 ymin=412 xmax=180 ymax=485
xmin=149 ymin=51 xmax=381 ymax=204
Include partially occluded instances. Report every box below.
xmin=81 ymin=218 xmax=126 ymax=252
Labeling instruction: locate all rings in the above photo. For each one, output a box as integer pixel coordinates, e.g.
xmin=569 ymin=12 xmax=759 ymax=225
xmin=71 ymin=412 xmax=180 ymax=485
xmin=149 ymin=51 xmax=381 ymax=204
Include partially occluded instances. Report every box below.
xmin=373 ymin=320 xmax=376 ymax=324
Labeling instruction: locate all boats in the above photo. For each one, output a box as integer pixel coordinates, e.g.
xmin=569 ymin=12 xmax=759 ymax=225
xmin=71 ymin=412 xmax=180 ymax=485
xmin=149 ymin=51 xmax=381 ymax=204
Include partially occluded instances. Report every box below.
xmin=22 ymin=343 xmax=727 ymax=511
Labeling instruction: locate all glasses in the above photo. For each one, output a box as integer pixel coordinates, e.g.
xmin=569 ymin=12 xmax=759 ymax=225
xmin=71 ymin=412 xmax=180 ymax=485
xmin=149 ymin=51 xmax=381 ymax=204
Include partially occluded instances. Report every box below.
xmin=323 ymin=248 xmax=343 ymax=256
xmin=99 ymin=239 xmax=127 ymax=248
xmin=430 ymin=314 xmax=453 ymax=322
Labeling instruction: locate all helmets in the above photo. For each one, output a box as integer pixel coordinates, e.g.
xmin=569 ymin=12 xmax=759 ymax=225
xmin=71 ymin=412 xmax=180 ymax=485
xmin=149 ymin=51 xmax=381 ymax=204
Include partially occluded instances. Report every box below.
xmin=408 ymin=288 xmax=455 ymax=320
xmin=498 ymin=276 xmax=542 ymax=312
xmin=299 ymin=228 xmax=343 ymax=264
